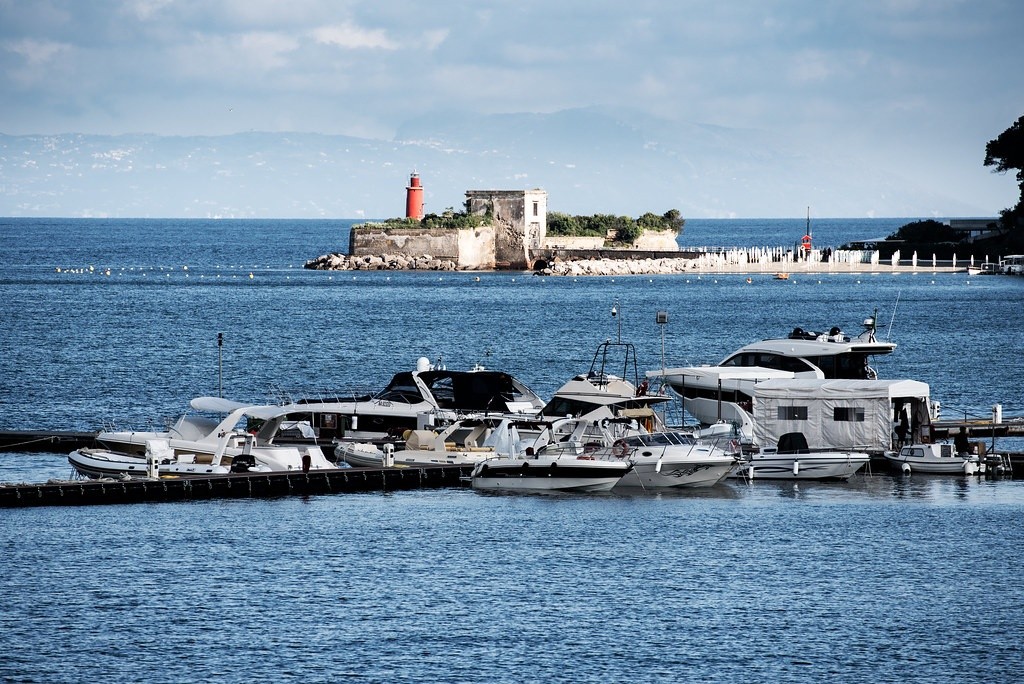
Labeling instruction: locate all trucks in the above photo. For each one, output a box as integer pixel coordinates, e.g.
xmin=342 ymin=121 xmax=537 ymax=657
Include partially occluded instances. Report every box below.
xmin=980 ymin=262 xmax=1004 ymax=275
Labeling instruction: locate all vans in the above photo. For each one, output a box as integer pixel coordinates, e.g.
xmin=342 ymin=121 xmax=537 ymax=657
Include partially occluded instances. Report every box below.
xmin=999 ymin=258 xmax=1024 ymax=275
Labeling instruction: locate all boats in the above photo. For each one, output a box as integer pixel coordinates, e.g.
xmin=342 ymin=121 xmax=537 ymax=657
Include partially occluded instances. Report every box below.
xmin=67 ymin=292 xmax=898 ymax=481
xmin=883 ymin=440 xmax=1014 ymax=477
xmin=458 ymin=440 xmax=639 ymax=495
xmin=727 ymin=444 xmax=872 ymax=483
